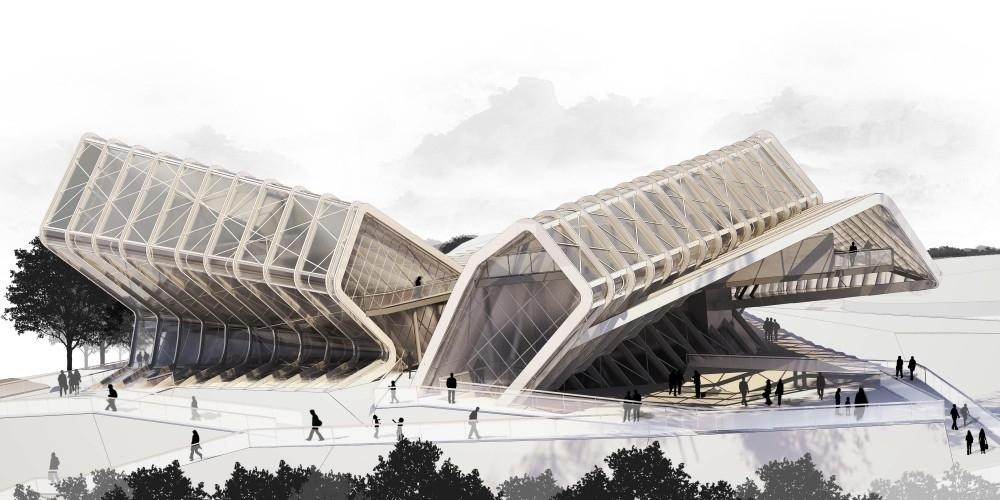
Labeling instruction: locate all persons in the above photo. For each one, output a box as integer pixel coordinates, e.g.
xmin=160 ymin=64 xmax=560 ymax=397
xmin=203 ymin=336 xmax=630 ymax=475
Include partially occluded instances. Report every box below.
xmin=136 ymin=351 xmax=143 ymax=369
xmin=848 ymin=242 xmax=857 ymax=266
xmin=388 ymin=381 xmax=400 ymax=404
xmin=692 ymin=370 xmax=701 ymax=398
xmin=961 ymin=404 xmax=970 ymax=426
xmin=631 ymin=389 xmax=641 ymax=422
xmin=854 ymin=388 xmax=869 ymax=420
xmin=480 ymin=359 xmax=487 ymax=383
xmin=623 ymin=390 xmax=632 ymax=422
xmin=835 ymin=388 xmax=840 ymax=415
xmin=896 ymin=356 xmax=904 ymax=379
xmin=48 ymin=452 xmax=59 ymax=486
xmin=73 ymin=368 xmax=82 ymax=395
xmin=978 ymin=428 xmax=986 ymax=454
xmin=908 ymin=356 xmax=916 ymax=380
xmin=191 ymin=396 xmax=200 ymax=420
xmin=468 ymin=407 xmax=481 ymax=439
xmin=305 ymin=409 xmax=325 ymax=441
xmin=966 ymin=430 xmax=974 ymax=455
xmin=667 ymin=367 xmax=684 ymax=396
xmin=105 ymin=384 xmax=118 ymax=411
xmin=775 ymin=379 xmax=783 ymax=406
xmin=863 ymin=240 xmax=873 ymax=266
xmin=446 ymin=373 xmax=457 ymax=405
xmin=845 ymin=397 xmax=851 ymax=415
xmin=816 ymin=372 xmax=826 ymax=400
xmin=765 ymin=379 xmax=773 ymax=405
xmin=473 ymin=360 xmax=480 ymax=383
xmin=392 ymin=417 xmax=404 ymax=440
xmin=373 ymin=415 xmax=380 ymax=438
xmin=406 ymin=352 xmax=413 ymax=379
xmin=411 ymin=275 xmax=423 ymax=297
xmin=56 ymin=370 xmax=69 ymax=397
xmin=143 ymin=352 xmax=150 ymax=366
xmin=763 ymin=317 xmax=781 ymax=342
xmin=738 ymin=377 xmax=749 ymax=406
xmin=950 ymin=403 xmax=960 ymax=430
xmin=189 ymin=430 xmax=203 ymax=460
xmin=67 ymin=369 xmax=75 ymax=395
